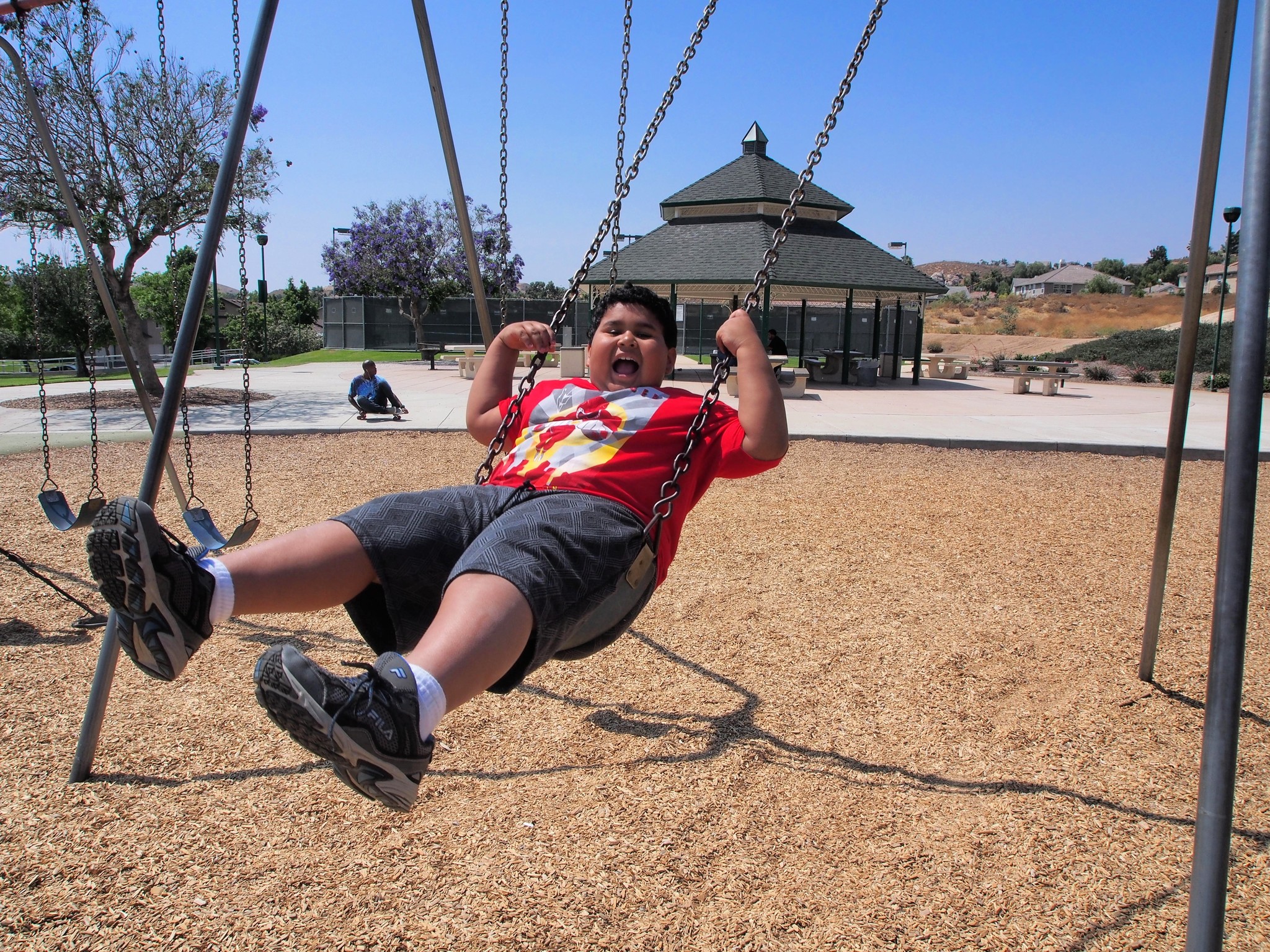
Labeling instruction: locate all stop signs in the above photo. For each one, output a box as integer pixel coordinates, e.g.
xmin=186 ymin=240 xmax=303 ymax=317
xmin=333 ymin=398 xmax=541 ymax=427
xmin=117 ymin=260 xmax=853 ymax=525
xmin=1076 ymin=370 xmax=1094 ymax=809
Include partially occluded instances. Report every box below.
xmin=204 ymin=347 xmax=213 ymax=356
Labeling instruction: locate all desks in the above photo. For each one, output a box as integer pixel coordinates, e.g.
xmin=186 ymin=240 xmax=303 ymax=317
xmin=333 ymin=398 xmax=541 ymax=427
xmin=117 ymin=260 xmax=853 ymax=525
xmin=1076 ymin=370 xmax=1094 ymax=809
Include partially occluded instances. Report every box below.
xmin=791 ymin=368 xmax=810 ymax=398
xmin=723 ymin=366 xmax=738 ymax=396
xmin=766 ymin=354 xmax=792 ymax=397
xmin=921 ymin=353 xmax=970 ymax=378
xmin=445 ymin=345 xmax=486 ymax=358
xmin=999 ymin=360 xmax=1079 ymax=375
xmin=521 ymin=343 xmax=561 ymax=366
xmin=581 ymin=344 xmax=589 ymax=368
xmin=878 ymin=352 xmax=902 ymax=378
xmin=560 ymin=347 xmax=585 ymax=377
xmin=819 ymin=349 xmax=865 ymax=382
xmin=804 ymin=358 xmax=828 ymax=381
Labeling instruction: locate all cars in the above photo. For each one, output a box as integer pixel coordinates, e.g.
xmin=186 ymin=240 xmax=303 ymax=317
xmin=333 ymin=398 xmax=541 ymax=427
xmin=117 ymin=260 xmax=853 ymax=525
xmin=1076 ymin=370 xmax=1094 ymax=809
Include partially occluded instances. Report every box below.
xmin=43 ymin=364 xmax=77 ymax=372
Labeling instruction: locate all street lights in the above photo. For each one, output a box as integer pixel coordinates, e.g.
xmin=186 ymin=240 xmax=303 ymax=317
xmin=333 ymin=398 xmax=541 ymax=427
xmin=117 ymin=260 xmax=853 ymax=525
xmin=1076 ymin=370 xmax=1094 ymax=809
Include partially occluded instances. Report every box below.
xmin=296 ymin=302 xmax=302 ymax=350
xmin=256 ymin=235 xmax=269 ymax=362
xmin=332 ymin=227 xmax=354 ymax=296
xmin=888 ymin=242 xmax=907 ymax=263
xmin=1208 ymin=206 xmax=1242 ymax=393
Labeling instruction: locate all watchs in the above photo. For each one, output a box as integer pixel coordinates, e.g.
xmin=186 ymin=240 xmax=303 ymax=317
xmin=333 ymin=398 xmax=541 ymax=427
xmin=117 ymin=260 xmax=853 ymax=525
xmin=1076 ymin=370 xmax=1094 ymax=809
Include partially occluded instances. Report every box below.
xmin=400 ymin=405 xmax=405 ymax=409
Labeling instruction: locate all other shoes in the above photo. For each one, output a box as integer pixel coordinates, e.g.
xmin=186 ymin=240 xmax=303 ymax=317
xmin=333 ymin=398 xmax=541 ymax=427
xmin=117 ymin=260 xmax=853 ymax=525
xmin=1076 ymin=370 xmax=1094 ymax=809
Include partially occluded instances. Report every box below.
xmin=387 ymin=406 xmax=401 ymax=414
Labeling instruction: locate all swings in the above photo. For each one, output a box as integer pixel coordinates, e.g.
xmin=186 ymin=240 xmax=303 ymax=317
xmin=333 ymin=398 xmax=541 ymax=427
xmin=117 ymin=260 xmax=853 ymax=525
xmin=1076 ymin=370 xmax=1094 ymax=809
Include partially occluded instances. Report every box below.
xmin=499 ymin=0 xmax=633 ymax=332
xmin=156 ymin=0 xmax=261 ymax=552
xmin=17 ymin=0 xmax=109 ymax=531
xmin=473 ymin=1 xmax=889 ymax=663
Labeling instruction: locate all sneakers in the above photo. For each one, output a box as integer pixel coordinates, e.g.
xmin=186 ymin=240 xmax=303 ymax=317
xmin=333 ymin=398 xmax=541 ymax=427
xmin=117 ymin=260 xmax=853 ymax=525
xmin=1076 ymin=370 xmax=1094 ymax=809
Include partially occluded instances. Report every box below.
xmin=85 ymin=495 xmax=216 ymax=682
xmin=251 ymin=644 xmax=436 ymax=814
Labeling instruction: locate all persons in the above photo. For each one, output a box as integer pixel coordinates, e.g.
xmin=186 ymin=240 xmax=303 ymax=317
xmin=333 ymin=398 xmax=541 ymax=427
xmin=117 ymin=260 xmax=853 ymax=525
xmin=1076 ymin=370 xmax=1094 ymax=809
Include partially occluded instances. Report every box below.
xmin=348 ymin=360 xmax=408 ymax=416
xmin=87 ymin=282 xmax=789 ymax=813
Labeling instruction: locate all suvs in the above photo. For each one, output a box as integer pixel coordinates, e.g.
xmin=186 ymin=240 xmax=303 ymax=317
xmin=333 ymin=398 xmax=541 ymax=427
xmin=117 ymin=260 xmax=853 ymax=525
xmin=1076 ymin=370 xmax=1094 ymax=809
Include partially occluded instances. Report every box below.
xmin=214 ymin=354 xmax=245 ymax=363
xmin=228 ymin=358 xmax=262 ymax=366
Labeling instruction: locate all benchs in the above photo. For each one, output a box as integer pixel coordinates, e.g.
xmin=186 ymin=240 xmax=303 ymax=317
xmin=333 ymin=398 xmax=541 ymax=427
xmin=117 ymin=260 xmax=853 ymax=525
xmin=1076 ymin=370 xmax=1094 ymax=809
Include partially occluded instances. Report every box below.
xmin=995 ymin=371 xmax=1079 ymax=396
xmin=455 ymin=357 xmax=484 ymax=378
xmin=516 ymin=351 xmax=560 ymax=367
xmin=440 ymin=355 xmax=486 ymax=371
xmin=910 ymin=361 xmax=979 ymax=379
